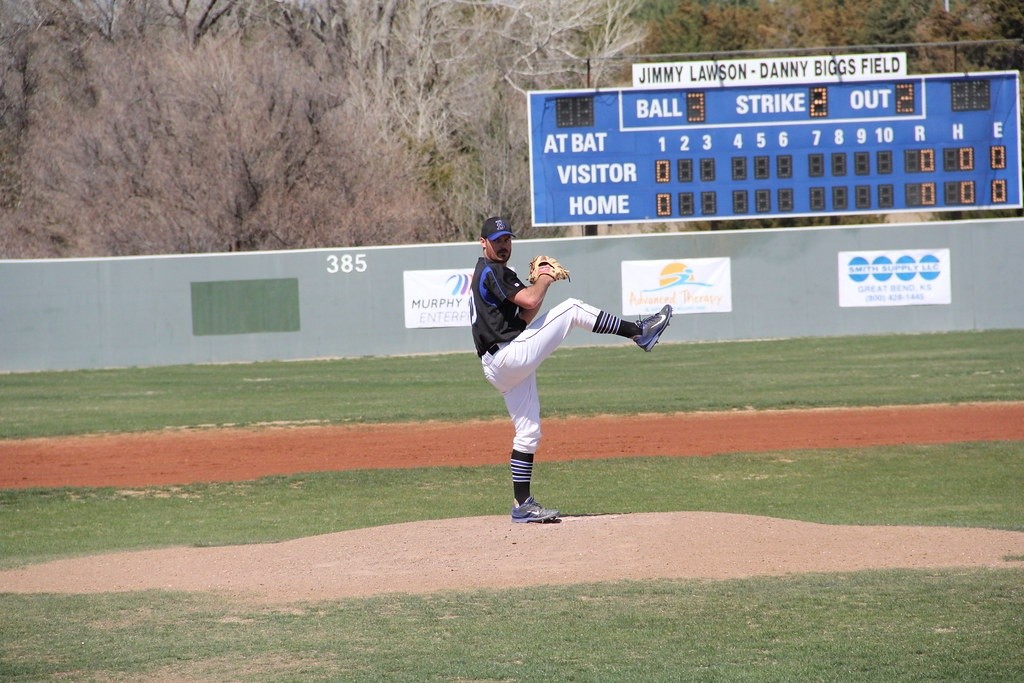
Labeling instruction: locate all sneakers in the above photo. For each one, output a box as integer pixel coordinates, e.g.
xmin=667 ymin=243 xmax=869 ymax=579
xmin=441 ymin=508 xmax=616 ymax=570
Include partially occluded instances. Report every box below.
xmin=633 ymin=304 xmax=674 ymax=352
xmin=511 ymin=494 xmax=560 ymax=523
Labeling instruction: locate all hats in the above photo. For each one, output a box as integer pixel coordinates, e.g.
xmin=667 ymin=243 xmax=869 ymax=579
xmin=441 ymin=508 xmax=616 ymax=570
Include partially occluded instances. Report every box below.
xmin=481 ymin=217 xmax=516 ymax=241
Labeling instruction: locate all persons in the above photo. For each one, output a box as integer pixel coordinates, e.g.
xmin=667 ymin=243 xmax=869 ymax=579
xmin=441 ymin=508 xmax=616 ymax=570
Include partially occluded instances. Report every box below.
xmin=470 ymin=217 xmax=674 ymax=522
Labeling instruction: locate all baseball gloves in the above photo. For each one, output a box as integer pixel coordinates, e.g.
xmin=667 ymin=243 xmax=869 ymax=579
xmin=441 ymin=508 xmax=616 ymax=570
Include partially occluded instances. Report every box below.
xmin=527 ymin=255 xmax=569 ymax=284
